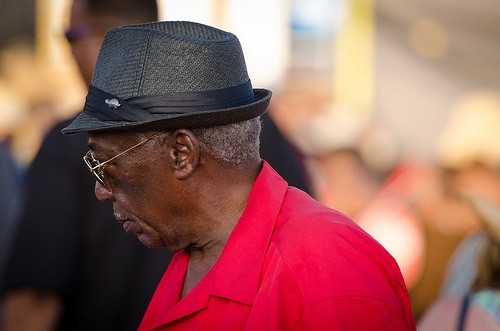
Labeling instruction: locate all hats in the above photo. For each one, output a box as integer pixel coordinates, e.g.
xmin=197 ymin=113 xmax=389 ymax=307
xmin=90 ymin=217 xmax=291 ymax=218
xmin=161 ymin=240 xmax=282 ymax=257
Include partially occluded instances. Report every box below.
xmin=63 ymin=23 xmax=273 ymax=134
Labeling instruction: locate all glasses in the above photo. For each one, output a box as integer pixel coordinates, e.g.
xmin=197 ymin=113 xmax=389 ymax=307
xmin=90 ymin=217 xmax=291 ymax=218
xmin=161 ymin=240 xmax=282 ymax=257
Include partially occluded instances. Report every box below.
xmin=83 ymin=128 xmax=193 ymax=193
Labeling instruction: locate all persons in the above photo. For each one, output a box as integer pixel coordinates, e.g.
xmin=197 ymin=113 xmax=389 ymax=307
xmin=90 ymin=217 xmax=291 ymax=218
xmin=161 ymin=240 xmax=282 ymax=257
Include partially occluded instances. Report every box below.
xmin=1 ymin=0 xmax=320 ymax=331
xmin=60 ymin=18 xmax=417 ymax=331
